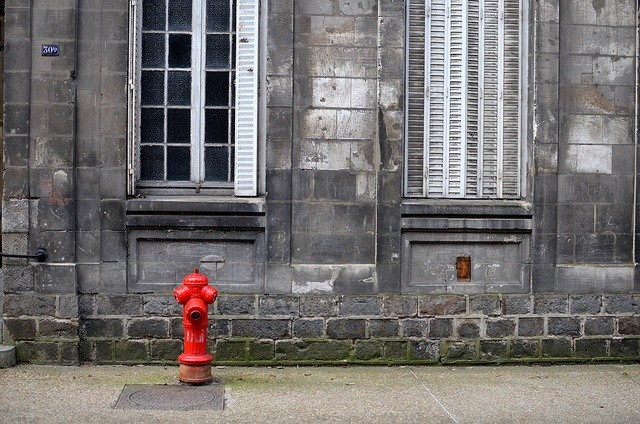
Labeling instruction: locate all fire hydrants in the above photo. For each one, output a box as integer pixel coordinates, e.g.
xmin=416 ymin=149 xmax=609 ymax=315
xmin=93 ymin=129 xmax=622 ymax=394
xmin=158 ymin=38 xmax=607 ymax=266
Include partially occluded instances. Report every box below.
xmin=173 ymin=267 xmax=218 ymax=386
